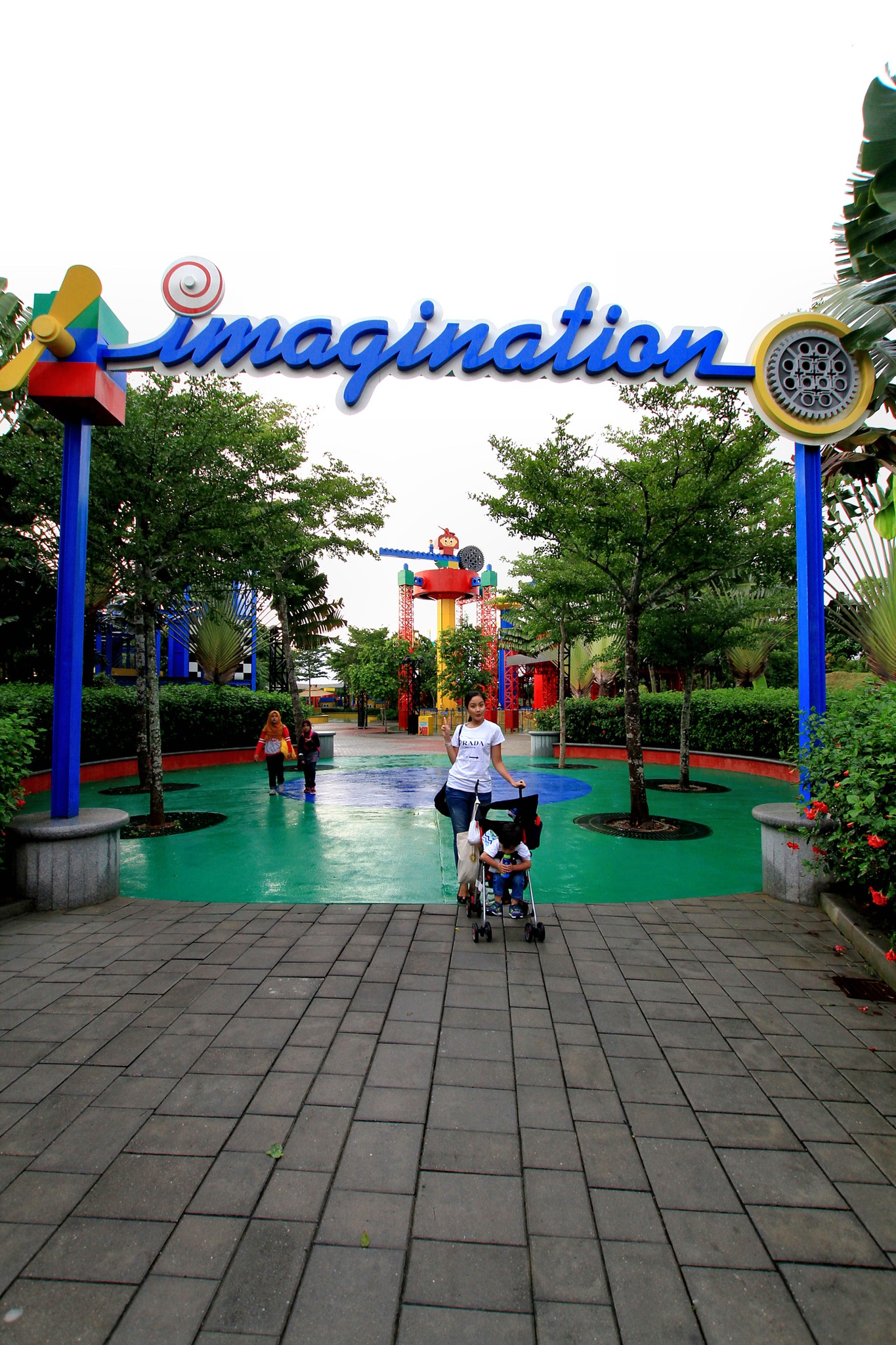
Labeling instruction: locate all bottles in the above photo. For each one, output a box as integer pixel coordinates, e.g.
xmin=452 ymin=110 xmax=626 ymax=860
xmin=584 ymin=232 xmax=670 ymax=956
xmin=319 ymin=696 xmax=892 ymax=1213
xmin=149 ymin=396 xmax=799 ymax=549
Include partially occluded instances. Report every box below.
xmin=501 ymin=854 xmax=511 ymax=878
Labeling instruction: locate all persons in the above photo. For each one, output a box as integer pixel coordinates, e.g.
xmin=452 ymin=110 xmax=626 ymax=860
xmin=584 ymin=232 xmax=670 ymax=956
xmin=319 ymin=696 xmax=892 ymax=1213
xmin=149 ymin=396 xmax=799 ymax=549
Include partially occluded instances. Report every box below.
xmin=255 ymin=710 xmax=292 ymax=794
xmin=297 ymin=720 xmax=320 ymax=794
xmin=442 ymin=691 xmax=526 ymax=904
xmin=428 ymin=540 xmax=434 ymax=553
xmin=480 ymin=821 xmax=531 ymax=918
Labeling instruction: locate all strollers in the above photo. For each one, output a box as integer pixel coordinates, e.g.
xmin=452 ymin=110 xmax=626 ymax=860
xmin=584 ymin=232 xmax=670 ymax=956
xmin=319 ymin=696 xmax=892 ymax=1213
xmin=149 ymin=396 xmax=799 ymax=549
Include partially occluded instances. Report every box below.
xmin=464 ymin=780 xmax=547 ymax=944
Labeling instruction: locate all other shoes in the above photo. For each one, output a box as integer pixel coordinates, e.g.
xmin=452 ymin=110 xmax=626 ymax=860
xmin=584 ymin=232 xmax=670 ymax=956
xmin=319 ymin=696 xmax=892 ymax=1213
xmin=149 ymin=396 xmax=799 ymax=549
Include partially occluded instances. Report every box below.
xmin=471 ymin=890 xmax=480 ymax=901
xmin=456 ymin=884 xmax=469 ymax=904
xmin=304 ymin=787 xmax=311 ymax=792
xmin=310 ymin=787 xmax=316 ymax=794
xmin=278 ymin=784 xmax=284 ymax=793
xmin=269 ymin=789 xmax=276 ymax=795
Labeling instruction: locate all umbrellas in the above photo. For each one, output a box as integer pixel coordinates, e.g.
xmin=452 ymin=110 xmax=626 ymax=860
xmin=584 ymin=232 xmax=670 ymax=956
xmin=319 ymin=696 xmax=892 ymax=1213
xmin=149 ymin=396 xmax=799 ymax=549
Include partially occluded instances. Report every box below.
xmin=298 ymin=686 xmax=334 ymax=708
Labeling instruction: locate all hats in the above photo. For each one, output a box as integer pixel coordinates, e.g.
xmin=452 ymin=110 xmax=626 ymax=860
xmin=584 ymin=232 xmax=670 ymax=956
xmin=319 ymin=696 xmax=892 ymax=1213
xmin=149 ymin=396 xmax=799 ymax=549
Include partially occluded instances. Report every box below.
xmin=302 ymin=720 xmax=310 ymax=727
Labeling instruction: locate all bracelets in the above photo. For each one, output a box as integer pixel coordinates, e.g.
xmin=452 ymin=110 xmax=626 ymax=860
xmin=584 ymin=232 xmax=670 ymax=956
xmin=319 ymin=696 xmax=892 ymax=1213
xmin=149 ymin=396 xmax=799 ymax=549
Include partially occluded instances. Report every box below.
xmin=445 ymin=740 xmax=452 ymax=747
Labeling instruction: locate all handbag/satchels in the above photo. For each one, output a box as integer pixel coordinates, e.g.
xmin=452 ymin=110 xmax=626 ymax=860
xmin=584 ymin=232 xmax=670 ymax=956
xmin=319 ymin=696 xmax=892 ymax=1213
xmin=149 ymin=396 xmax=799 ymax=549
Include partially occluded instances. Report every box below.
xmin=467 ymin=802 xmax=481 ymax=846
xmin=281 ymin=738 xmax=296 ymax=759
xmin=434 ymin=779 xmax=452 ymax=820
xmin=458 ymin=800 xmax=480 ymax=884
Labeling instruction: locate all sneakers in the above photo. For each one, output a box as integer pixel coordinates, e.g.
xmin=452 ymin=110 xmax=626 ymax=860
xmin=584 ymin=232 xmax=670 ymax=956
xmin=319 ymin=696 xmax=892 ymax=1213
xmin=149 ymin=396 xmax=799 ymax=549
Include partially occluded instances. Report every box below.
xmin=508 ymin=905 xmax=523 ymax=918
xmin=485 ymin=903 xmax=502 ymax=916
